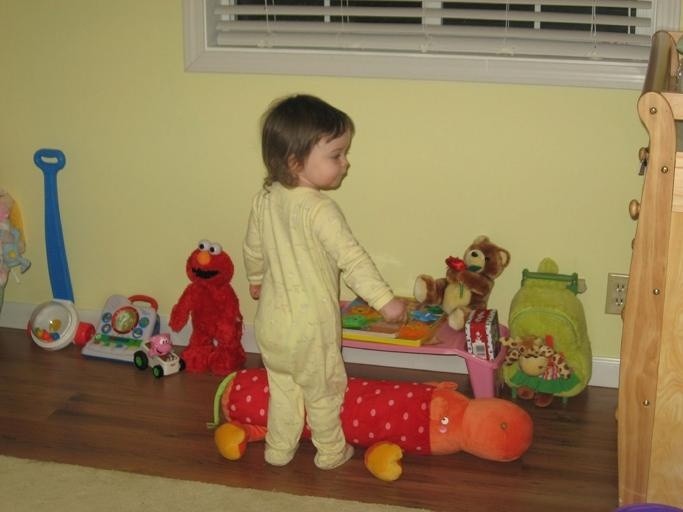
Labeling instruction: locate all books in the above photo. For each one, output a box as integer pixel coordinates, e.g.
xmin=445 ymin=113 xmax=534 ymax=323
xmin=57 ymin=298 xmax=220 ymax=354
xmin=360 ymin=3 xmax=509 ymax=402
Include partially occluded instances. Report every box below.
xmin=338 ymin=298 xmax=447 ymax=347
xmin=339 ymin=294 xmax=419 ymax=339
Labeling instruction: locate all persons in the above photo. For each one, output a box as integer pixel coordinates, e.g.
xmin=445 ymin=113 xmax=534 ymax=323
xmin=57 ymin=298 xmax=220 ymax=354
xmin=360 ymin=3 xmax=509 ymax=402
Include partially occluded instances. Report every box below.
xmin=0 ymin=191 xmax=34 ymax=289
xmin=242 ymin=92 xmax=410 ymax=473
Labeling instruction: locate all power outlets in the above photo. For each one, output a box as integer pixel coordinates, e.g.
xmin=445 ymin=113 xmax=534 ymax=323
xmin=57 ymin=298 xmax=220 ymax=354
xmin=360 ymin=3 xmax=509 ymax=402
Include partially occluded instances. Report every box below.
xmin=604 ymin=273 xmax=630 ymax=315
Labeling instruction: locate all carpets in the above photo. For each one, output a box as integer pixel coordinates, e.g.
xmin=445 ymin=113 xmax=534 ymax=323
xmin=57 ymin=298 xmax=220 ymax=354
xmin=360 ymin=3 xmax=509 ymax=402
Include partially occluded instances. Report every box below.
xmin=1 ymin=454 xmax=446 ymax=512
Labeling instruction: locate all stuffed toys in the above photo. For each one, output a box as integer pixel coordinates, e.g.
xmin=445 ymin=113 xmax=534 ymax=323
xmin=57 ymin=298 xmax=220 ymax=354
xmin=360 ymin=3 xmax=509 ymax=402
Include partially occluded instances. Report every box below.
xmin=497 ymin=336 xmax=571 ymax=408
xmin=205 ymin=364 xmax=536 ymax=484
xmin=414 ymin=232 xmax=513 ymax=334
xmin=169 ymin=236 xmax=251 ymax=381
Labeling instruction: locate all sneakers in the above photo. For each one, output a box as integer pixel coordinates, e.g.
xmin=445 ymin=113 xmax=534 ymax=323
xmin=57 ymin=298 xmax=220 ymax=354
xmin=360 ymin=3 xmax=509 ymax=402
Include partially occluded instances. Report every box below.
xmin=262 ymin=444 xmax=301 ymax=467
xmin=313 ymin=442 xmax=353 ymax=469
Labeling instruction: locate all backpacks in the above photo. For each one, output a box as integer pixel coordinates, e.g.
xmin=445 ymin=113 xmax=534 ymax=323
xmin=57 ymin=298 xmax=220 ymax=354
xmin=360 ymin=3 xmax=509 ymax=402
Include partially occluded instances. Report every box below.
xmin=502 ymin=257 xmax=592 ymax=398
